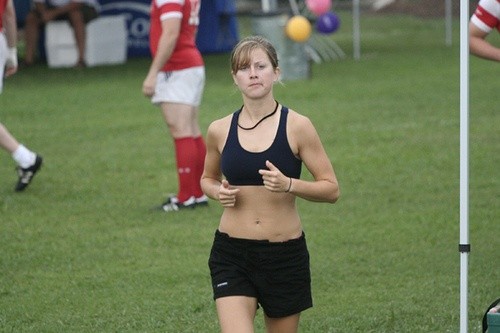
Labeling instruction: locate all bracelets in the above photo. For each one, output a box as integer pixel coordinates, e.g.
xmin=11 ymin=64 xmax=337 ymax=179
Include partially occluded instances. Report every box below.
xmin=285 ymin=178 xmax=292 ymax=193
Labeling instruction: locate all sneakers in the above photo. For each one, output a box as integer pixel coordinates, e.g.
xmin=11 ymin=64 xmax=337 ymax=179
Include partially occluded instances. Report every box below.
xmin=168 ymin=192 xmax=209 ymax=206
xmin=162 ymin=195 xmax=197 ymax=212
xmin=14 ymin=153 xmax=43 ymax=192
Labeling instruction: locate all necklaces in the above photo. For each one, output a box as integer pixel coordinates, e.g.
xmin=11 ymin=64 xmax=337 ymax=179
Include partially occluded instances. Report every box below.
xmin=236 ymin=99 xmax=279 ymax=130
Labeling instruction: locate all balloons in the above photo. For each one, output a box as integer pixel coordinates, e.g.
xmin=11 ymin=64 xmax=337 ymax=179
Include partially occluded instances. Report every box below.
xmin=285 ymin=0 xmax=340 ymax=42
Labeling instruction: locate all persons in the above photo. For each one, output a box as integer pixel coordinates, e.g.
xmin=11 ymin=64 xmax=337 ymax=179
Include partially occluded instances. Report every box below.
xmin=0 ymin=0 xmax=42 ymax=193
xmin=141 ymin=0 xmax=209 ymax=212
xmin=200 ymin=37 xmax=340 ymax=333
xmin=19 ymin=0 xmax=103 ymax=74
xmin=468 ymin=0 xmax=500 ymax=62
